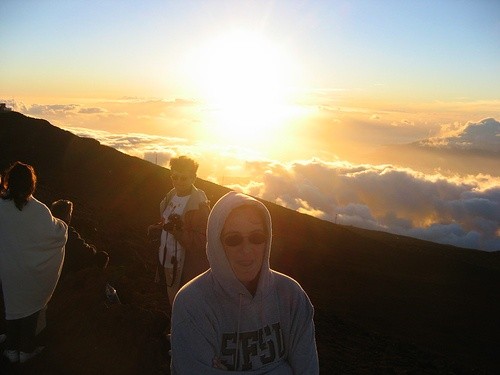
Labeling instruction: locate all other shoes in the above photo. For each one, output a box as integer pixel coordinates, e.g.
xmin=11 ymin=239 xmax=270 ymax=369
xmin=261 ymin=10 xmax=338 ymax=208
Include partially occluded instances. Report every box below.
xmin=0 ymin=335 xmax=6 ymax=344
xmin=5 ymin=350 xmax=19 ymax=361
xmin=20 ymin=347 xmax=46 ymax=362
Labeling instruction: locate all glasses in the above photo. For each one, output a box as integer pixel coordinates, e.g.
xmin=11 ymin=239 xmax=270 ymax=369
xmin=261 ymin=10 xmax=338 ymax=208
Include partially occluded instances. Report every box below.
xmin=172 ymin=174 xmax=191 ymax=181
xmin=220 ymin=233 xmax=267 ymax=247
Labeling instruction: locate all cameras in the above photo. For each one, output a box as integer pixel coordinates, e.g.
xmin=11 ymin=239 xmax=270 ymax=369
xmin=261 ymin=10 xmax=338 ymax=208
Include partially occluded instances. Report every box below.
xmin=163 ymin=214 xmax=183 ymax=231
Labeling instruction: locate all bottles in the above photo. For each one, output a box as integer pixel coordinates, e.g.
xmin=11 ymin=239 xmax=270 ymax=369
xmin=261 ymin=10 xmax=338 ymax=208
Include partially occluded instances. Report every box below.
xmin=103 ymin=281 xmax=122 ymax=305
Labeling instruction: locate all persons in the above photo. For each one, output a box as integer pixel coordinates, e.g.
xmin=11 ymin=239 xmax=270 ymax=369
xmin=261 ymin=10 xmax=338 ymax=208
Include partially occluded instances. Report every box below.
xmin=170 ymin=191 xmax=320 ymax=375
xmin=51 ymin=199 xmax=118 ymax=297
xmin=146 ymin=154 xmax=211 ymax=357
xmin=0 ymin=162 xmax=69 ymax=361
